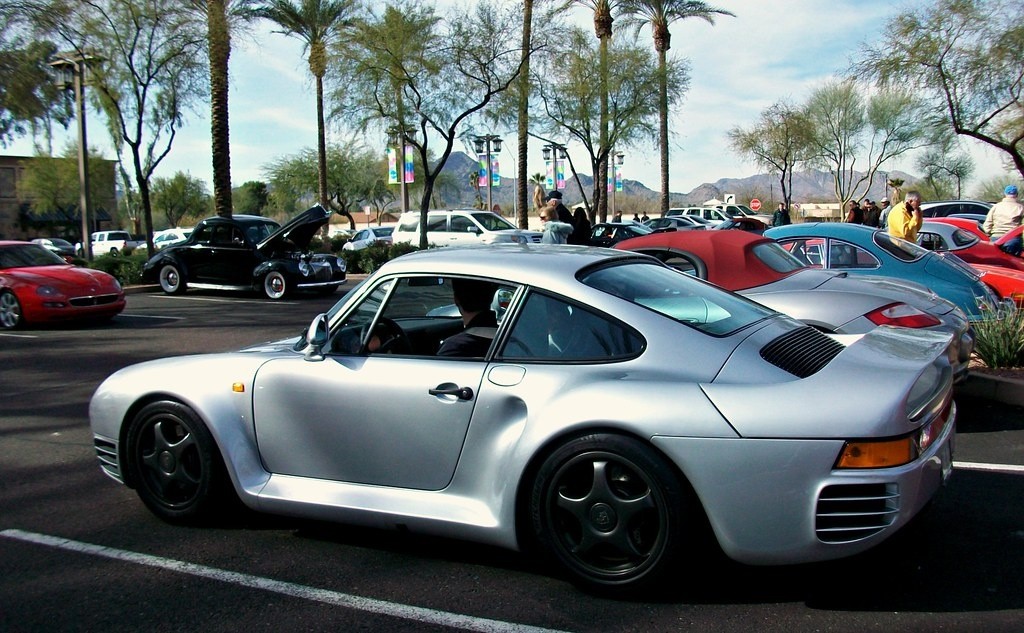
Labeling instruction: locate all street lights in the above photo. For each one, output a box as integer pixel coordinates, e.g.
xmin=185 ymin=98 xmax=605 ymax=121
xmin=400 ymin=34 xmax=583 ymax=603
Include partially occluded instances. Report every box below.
xmin=386 ymin=122 xmax=419 ymax=214
xmin=471 ymin=134 xmax=504 ymax=212
xmin=50 ymin=47 xmax=112 ymax=262
xmin=541 ymin=142 xmax=569 ymax=191
xmin=609 ymin=144 xmax=625 ymax=218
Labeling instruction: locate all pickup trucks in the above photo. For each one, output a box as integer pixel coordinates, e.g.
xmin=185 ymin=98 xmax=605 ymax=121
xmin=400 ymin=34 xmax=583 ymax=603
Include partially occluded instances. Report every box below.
xmin=75 ymin=230 xmax=145 ymax=257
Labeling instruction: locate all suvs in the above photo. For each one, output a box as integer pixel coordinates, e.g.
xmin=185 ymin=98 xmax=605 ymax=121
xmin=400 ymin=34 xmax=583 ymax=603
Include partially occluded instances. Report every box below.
xmin=712 ymin=203 xmax=774 ymax=227
xmin=390 ymin=206 xmax=544 ymax=249
xmin=665 ymin=205 xmax=735 ymax=228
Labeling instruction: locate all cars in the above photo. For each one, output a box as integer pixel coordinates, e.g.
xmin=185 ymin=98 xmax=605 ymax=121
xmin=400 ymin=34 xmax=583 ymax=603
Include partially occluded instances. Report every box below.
xmin=670 ymin=214 xmax=717 ymax=231
xmin=340 ymin=225 xmax=394 ymax=253
xmin=712 ymin=218 xmax=770 ymax=235
xmin=780 ymin=200 xmax=1022 ymax=306
xmin=642 ymin=217 xmax=706 ymax=233
xmin=589 ymin=222 xmax=654 ymax=247
xmin=138 ymin=226 xmax=195 ymax=252
xmin=33 ymin=237 xmax=76 ymax=256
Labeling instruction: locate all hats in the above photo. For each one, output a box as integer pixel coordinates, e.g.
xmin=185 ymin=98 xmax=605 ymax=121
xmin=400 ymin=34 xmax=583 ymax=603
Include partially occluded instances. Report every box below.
xmin=881 ymin=198 xmax=889 ymax=202
xmin=1005 ymin=185 xmax=1017 ymax=195
xmin=542 ymin=191 xmax=562 ymax=200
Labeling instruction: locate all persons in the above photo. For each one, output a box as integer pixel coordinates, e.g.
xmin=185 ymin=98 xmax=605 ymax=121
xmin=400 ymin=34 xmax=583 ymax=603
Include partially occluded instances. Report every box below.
xmin=772 ymin=203 xmax=790 ymax=227
xmin=612 ymin=210 xmax=622 ymax=223
xmin=888 ymin=191 xmax=923 ymax=244
xmin=368 ymin=278 xmax=500 ymax=357
xmin=641 ymin=211 xmax=649 ymax=223
xmin=545 ymin=300 xmax=570 ymax=357
xmin=983 ymin=185 xmax=1024 ymax=257
xmin=845 ymin=197 xmax=891 ymax=229
xmin=633 ymin=211 xmax=640 ymax=223
xmin=541 ymin=191 xmax=592 ymax=246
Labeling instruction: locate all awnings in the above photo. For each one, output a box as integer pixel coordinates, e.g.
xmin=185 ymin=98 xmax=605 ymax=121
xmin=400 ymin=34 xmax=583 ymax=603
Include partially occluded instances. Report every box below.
xmin=21 ymin=204 xmax=112 ymax=221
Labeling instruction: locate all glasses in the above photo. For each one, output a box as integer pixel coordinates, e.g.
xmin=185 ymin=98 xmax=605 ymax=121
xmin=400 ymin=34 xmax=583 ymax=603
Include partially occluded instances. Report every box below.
xmin=540 ymin=216 xmax=549 ymax=220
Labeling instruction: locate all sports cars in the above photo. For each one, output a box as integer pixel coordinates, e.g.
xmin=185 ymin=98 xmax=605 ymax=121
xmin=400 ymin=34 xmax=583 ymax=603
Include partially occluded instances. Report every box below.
xmin=81 ymin=239 xmax=961 ymax=598
xmin=138 ymin=200 xmax=351 ymax=303
xmin=682 ymin=222 xmax=1017 ymax=339
xmin=417 ymin=229 xmax=976 ymax=444
xmin=0 ymin=241 xmax=128 ymax=331
